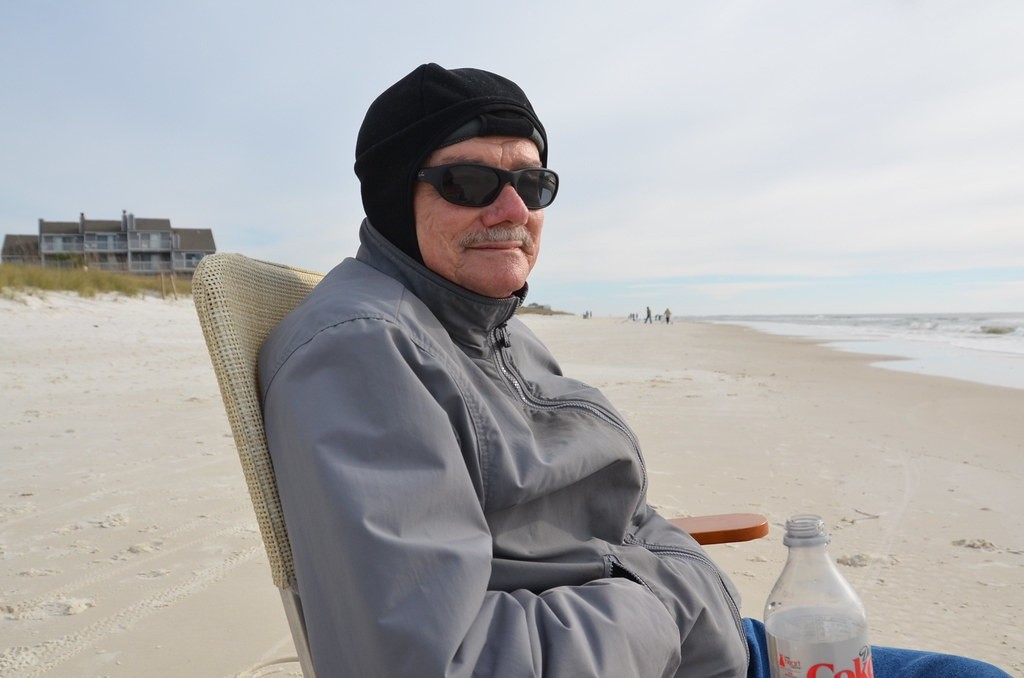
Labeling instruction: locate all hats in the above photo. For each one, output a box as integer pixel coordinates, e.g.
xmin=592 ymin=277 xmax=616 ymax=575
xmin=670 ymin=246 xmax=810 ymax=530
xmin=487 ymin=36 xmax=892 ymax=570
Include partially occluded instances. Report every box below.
xmin=354 ymin=63 xmax=549 ymax=266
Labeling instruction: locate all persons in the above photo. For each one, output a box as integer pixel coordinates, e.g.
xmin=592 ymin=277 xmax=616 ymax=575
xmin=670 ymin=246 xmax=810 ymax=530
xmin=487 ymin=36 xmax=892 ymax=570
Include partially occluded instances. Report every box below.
xmin=645 ymin=307 xmax=653 ymax=324
xmin=257 ymin=63 xmax=1015 ymax=678
xmin=664 ymin=308 xmax=671 ymax=324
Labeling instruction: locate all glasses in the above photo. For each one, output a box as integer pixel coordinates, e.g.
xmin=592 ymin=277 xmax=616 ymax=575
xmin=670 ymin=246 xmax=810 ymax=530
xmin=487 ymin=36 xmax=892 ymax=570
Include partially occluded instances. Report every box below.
xmin=415 ymin=163 xmax=561 ymax=210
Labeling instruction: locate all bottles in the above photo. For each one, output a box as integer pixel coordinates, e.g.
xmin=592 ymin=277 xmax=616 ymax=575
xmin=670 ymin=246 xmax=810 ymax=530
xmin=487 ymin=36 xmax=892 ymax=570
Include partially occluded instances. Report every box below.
xmin=762 ymin=513 xmax=874 ymax=678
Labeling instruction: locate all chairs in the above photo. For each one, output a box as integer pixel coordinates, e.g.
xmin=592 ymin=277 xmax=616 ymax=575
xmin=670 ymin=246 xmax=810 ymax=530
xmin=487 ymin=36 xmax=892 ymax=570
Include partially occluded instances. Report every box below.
xmin=188 ymin=252 xmax=770 ymax=678
